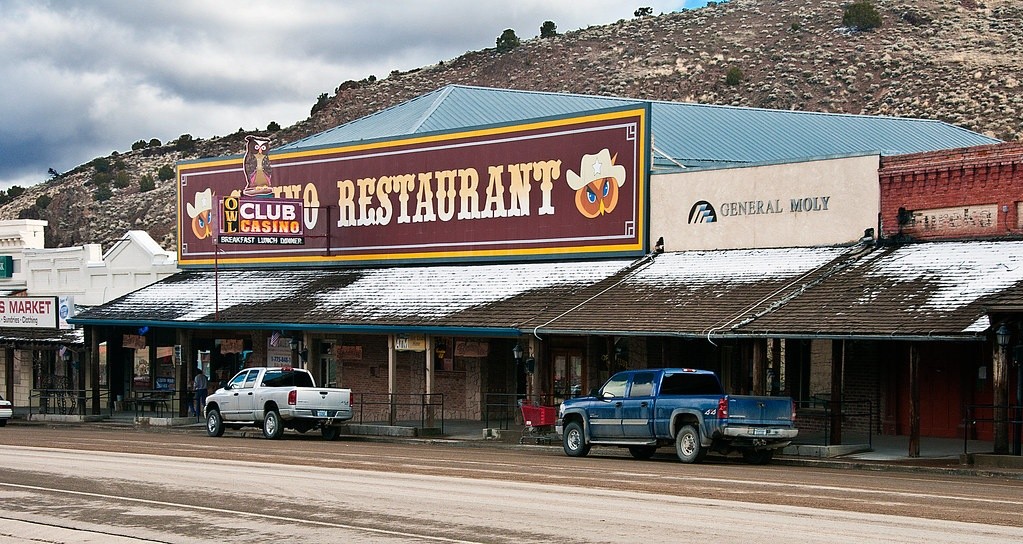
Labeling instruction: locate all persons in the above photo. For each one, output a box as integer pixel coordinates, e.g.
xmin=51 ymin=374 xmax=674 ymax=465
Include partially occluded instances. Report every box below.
xmin=194 ymin=369 xmax=209 ymax=417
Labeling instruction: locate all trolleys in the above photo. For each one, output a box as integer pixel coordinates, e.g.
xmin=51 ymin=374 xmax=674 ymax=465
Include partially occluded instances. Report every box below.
xmin=516 ymin=399 xmax=557 ymax=446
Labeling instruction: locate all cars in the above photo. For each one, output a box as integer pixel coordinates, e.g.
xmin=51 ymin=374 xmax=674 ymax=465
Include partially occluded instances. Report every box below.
xmin=0 ymin=395 xmax=12 ymax=427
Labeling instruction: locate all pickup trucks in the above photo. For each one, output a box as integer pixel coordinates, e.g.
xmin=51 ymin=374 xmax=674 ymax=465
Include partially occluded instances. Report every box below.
xmin=553 ymin=368 xmax=800 ymax=464
xmin=203 ymin=366 xmax=354 ymax=441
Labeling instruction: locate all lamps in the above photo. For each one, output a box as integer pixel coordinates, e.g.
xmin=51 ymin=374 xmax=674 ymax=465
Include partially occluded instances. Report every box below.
xmin=513 ymin=342 xmax=524 ymax=366
xmin=994 ymin=322 xmax=1010 ymax=355
xmin=289 ymin=336 xmax=299 ymax=353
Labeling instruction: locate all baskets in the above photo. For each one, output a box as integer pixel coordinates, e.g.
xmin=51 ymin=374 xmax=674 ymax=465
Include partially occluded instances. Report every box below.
xmin=521 ymin=406 xmax=556 ymax=426
